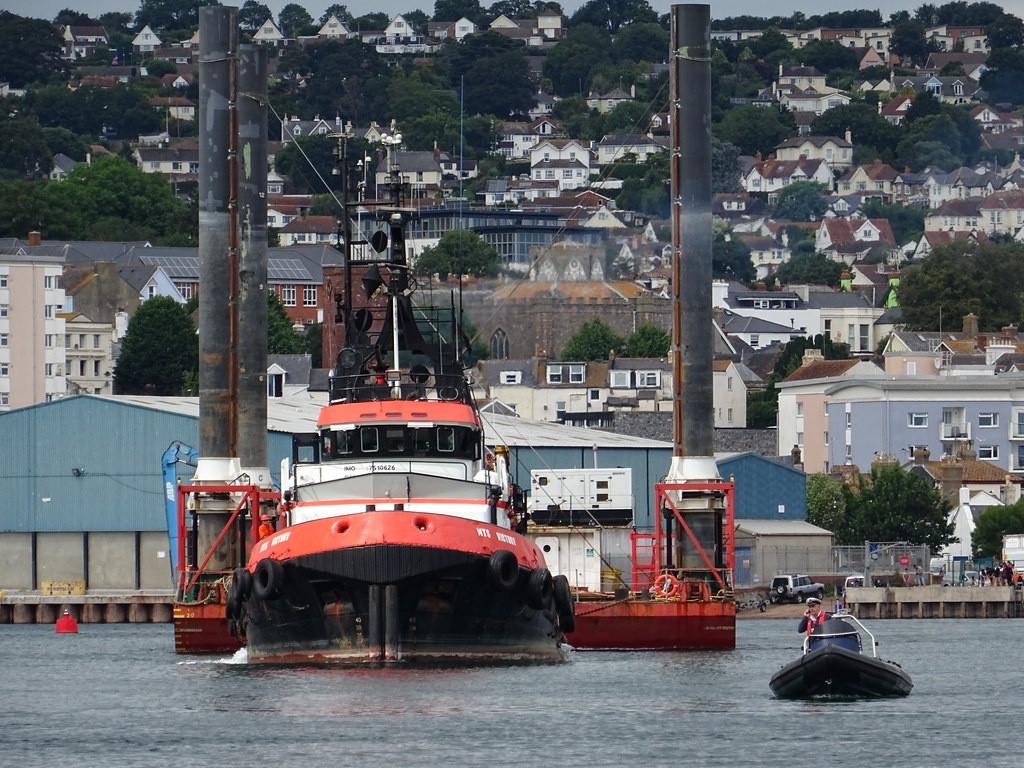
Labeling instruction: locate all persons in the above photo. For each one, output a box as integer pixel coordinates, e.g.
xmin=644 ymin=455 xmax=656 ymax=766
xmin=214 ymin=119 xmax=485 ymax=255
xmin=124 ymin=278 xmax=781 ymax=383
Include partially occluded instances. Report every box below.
xmin=913 ymin=565 xmax=925 ymax=586
xmin=279 ymin=491 xmax=297 ymax=528
xmin=797 ymin=598 xmax=831 ymax=639
xmin=936 ymin=558 xmax=1024 ymax=591
xmin=259 ymin=514 xmax=274 ymax=539
xmin=902 ymin=568 xmax=913 ymax=585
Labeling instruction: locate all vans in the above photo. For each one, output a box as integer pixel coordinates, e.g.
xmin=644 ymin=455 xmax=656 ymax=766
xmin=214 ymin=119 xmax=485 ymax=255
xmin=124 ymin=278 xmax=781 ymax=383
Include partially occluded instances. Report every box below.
xmin=941 ymin=571 xmax=991 ymax=588
xmin=844 ymin=575 xmax=864 ymax=589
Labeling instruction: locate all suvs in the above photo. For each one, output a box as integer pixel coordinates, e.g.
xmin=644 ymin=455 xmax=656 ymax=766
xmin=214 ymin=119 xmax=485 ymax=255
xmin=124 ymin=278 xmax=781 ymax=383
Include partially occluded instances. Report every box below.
xmin=768 ymin=574 xmax=824 ymax=604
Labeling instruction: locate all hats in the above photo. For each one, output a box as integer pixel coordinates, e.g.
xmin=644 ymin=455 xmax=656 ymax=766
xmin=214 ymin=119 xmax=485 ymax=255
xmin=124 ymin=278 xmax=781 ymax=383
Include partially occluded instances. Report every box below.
xmin=806 ymin=598 xmax=823 ymax=606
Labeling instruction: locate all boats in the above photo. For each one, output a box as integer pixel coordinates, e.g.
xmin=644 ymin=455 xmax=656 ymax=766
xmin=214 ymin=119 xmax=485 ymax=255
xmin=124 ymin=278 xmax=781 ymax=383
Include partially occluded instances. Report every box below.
xmin=768 ymin=615 xmax=914 ymax=702
xmin=170 ymin=6 xmax=743 ymax=657
xmin=227 ymin=124 xmax=575 ymax=668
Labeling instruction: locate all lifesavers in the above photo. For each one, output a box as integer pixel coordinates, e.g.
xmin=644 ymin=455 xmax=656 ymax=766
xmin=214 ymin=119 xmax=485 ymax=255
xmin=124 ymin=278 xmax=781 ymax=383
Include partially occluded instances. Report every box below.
xmin=654 ymin=575 xmax=679 ymax=598
